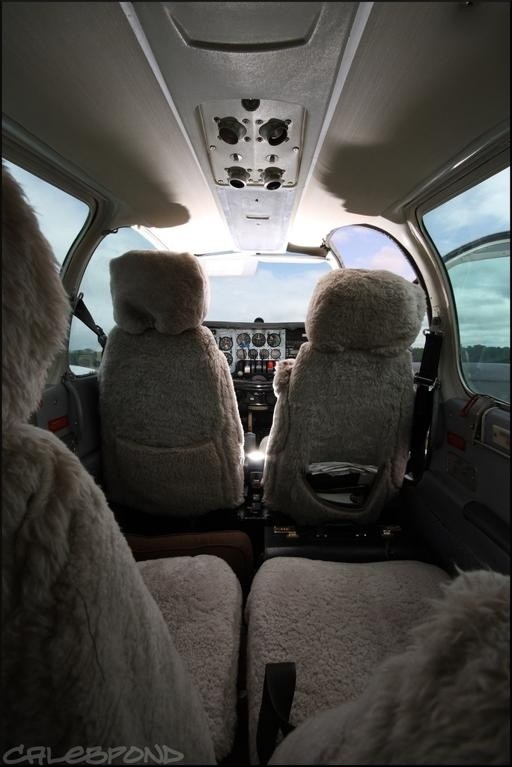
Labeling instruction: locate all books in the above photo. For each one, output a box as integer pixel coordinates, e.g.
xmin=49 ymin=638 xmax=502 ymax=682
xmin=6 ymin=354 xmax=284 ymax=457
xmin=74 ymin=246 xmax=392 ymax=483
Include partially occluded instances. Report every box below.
xmin=304 ymin=459 xmax=379 ymax=508
